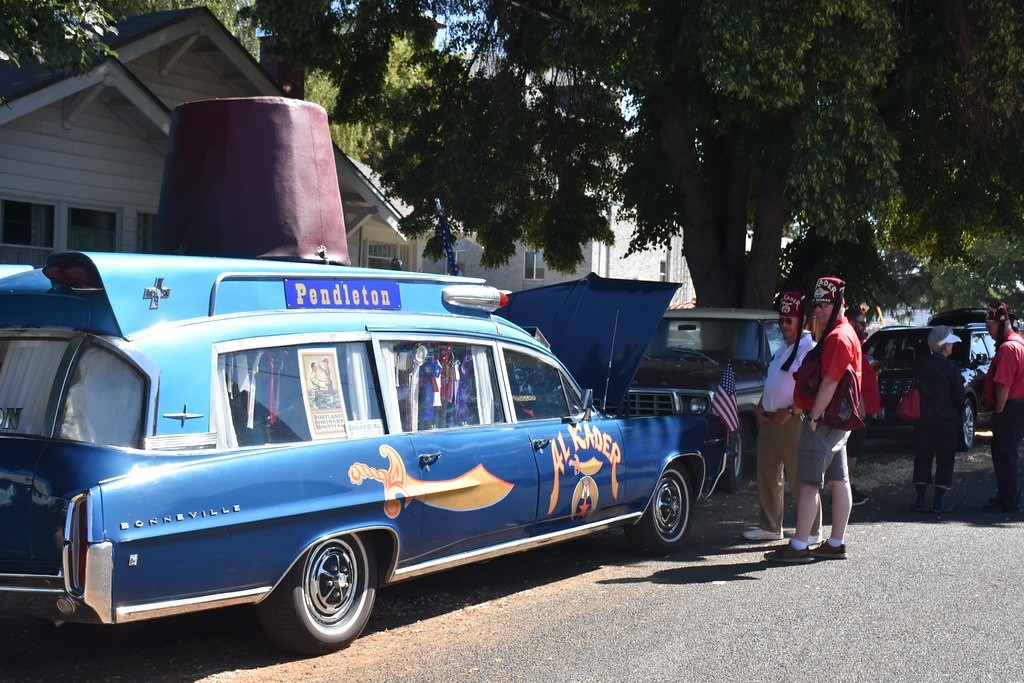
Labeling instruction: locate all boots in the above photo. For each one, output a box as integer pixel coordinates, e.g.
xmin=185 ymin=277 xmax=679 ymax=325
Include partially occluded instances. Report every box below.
xmin=932 ymin=483 xmax=954 ymax=512
xmin=913 ymin=482 xmax=927 ymax=511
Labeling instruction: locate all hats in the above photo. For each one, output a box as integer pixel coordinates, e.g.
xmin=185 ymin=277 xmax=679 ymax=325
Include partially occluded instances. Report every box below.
xmin=937 ymin=333 xmax=962 ymax=347
xmin=986 ymin=300 xmax=1009 ymax=323
xmin=812 ymin=275 xmax=845 ymax=302
xmin=778 ymin=290 xmax=807 ymax=317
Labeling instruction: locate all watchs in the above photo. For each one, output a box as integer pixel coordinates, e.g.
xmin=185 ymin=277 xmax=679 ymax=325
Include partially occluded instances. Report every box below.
xmin=788 ymin=406 xmax=795 ymax=416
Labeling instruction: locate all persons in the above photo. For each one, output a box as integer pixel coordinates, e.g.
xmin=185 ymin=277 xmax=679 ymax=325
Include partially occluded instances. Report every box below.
xmin=982 ymin=301 xmax=1024 ymax=508
xmin=910 ymin=325 xmax=966 ymax=513
xmin=763 ymin=277 xmax=888 ymax=564
xmin=743 ymin=286 xmax=822 ymax=545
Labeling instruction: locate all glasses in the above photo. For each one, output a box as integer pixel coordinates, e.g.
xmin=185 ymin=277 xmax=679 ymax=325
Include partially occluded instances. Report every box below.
xmin=811 ymin=301 xmax=833 ymax=310
xmin=852 ymin=319 xmax=866 ymax=328
xmin=778 ymin=317 xmax=799 ymax=326
xmin=986 ymin=320 xmax=1000 ymax=329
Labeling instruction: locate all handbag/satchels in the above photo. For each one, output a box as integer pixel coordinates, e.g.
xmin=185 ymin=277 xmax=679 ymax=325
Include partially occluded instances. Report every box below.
xmin=795 ymin=349 xmax=866 ymax=430
xmin=895 ymin=389 xmax=920 ymax=421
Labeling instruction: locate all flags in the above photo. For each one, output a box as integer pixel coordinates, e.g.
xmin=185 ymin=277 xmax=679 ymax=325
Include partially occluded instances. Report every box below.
xmin=711 ymin=363 xmax=740 ymax=432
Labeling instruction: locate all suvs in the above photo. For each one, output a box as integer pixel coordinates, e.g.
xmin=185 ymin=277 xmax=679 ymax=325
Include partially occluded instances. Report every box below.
xmin=606 ymin=306 xmax=790 ymax=495
xmin=844 ymin=307 xmax=1024 ymax=452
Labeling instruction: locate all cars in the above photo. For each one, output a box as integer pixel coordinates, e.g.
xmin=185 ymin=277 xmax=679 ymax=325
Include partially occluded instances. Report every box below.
xmin=1 ymin=248 xmax=737 ymax=658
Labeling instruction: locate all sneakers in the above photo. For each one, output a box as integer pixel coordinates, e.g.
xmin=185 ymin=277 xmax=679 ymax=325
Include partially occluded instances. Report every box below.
xmin=806 ymin=534 xmax=822 ymax=545
xmin=809 ymin=538 xmax=848 ymax=559
xmin=742 ymin=527 xmax=784 ymax=540
xmin=764 ymin=541 xmax=811 ymax=562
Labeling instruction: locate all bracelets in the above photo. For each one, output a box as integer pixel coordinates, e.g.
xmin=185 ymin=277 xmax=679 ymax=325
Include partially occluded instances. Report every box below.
xmin=807 ymin=416 xmax=819 ymax=423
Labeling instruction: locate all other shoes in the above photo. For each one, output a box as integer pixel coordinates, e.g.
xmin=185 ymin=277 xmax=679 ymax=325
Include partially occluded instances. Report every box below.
xmin=984 ymin=497 xmax=1019 ymax=514
xmin=827 ymin=483 xmax=868 ymax=505
